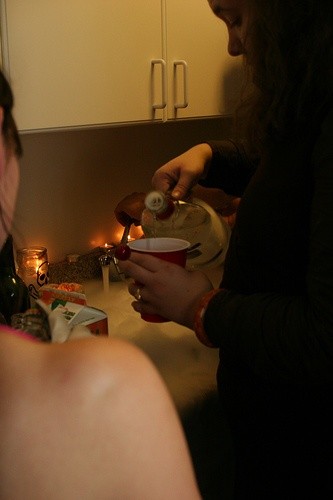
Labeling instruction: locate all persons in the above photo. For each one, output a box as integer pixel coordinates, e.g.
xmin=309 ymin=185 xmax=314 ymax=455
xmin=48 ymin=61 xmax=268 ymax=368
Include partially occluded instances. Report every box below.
xmin=0 ymin=71 xmax=201 ymax=500
xmin=118 ymin=1 xmax=333 ymax=499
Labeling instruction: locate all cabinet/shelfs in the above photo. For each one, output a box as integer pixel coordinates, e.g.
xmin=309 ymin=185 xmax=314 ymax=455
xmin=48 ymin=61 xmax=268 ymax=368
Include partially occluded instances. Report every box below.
xmin=0 ymin=0 xmax=246 ymax=135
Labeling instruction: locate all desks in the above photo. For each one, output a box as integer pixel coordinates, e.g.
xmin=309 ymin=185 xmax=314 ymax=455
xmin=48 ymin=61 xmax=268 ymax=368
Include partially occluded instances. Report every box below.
xmin=80 ymin=269 xmax=223 ymax=416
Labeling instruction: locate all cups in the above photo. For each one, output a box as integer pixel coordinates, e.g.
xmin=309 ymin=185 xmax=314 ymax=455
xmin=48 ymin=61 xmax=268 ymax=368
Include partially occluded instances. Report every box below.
xmin=127 ymin=237 xmax=191 ymax=323
xmin=15 ymin=246 xmax=53 ymax=297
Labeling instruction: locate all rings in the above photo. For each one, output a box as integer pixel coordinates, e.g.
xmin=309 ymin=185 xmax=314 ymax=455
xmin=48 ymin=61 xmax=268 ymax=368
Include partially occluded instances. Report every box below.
xmin=134 ymin=288 xmax=146 ymax=304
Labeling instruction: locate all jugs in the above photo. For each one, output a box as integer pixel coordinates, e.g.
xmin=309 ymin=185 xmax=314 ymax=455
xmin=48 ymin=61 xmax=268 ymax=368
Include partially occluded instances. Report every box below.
xmin=139 ymin=190 xmax=231 ymax=272
